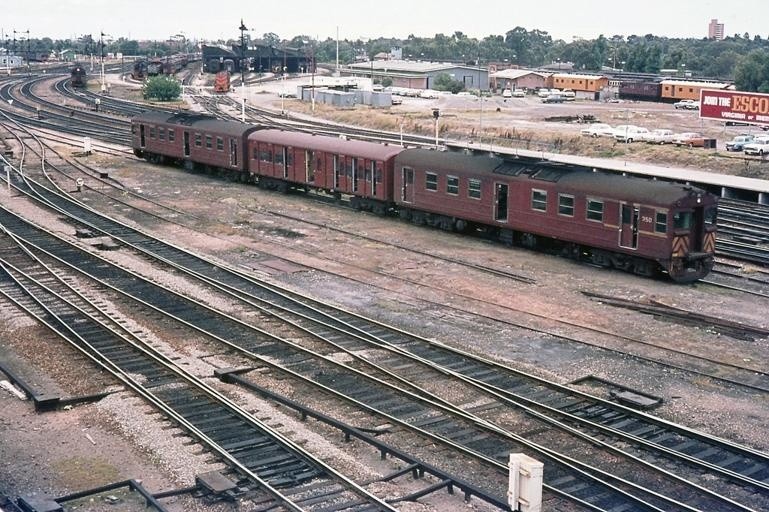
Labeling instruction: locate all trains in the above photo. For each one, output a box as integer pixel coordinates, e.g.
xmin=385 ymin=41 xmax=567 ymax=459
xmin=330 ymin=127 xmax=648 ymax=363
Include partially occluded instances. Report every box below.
xmin=71 ymin=66 xmax=86 ymax=88
xmin=615 ymin=78 xmax=739 ymax=101
xmin=129 ymin=112 xmax=717 ymax=284
xmin=148 ymin=52 xmax=202 ymax=78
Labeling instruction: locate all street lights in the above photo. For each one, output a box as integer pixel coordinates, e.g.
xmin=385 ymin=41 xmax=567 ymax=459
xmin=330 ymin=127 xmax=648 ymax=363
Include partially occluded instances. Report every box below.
xmin=421 ymin=51 xmax=426 ymax=62
xmin=608 ymin=56 xmax=616 ymax=69
xmin=618 ymin=60 xmax=627 ymax=98
xmin=275 ymin=66 xmax=290 ymax=115
xmin=301 ymin=36 xmax=315 ymax=111
xmin=406 ymin=53 xmax=412 ymax=61
xmin=681 ymin=60 xmax=687 ymax=78
xmin=567 ymin=60 xmax=575 ymax=75
xmin=513 ymin=54 xmax=521 ymax=71
xmin=553 ymin=58 xmax=564 ymax=74
xmin=249 ymin=59 xmax=263 ymax=93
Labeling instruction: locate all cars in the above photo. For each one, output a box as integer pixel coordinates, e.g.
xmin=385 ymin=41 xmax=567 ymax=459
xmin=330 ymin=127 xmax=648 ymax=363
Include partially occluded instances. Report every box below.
xmin=372 ymin=84 xmax=443 ymax=105
xmin=502 ymin=88 xmax=768 ymax=157
